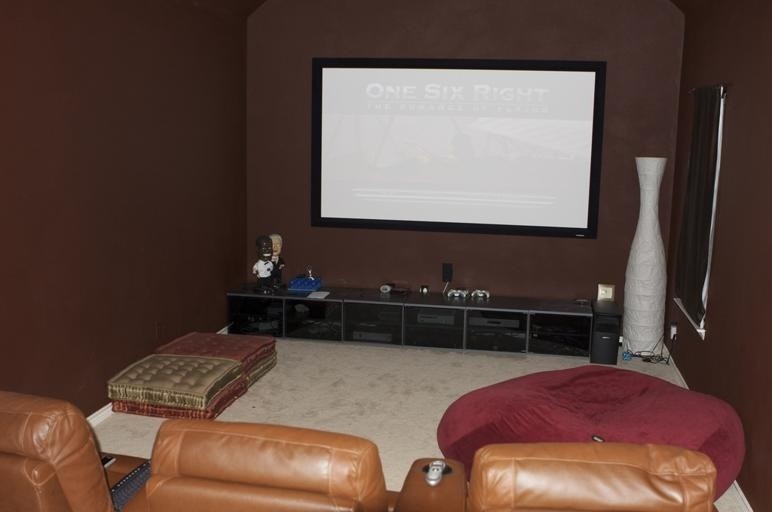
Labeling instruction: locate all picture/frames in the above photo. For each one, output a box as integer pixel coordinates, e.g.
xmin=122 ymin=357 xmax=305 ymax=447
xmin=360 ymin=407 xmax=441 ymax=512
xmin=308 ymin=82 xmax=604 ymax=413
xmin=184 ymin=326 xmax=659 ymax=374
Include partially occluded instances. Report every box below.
xmin=597 ymin=283 xmax=616 ymax=302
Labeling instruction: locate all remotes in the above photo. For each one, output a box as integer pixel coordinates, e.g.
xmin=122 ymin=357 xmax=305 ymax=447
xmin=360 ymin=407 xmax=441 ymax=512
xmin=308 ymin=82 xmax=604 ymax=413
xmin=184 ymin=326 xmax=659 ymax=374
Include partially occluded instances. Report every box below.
xmin=426 ymin=460 xmax=447 ymax=485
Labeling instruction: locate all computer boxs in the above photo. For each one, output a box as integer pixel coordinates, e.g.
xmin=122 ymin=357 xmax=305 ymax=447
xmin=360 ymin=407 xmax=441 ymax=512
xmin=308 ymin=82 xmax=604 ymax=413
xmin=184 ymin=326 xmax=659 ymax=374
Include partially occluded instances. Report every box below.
xmin=590 ymin=300 xmax=621 ymax=364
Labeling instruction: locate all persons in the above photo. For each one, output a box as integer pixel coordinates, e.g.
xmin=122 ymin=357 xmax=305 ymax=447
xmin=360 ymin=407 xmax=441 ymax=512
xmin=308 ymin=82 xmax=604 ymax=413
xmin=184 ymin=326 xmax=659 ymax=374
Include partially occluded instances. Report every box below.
xmin=252 ymin=234 xmax=274 ymax=295
xmin=269 ymin=233 xmax=285 ymax=290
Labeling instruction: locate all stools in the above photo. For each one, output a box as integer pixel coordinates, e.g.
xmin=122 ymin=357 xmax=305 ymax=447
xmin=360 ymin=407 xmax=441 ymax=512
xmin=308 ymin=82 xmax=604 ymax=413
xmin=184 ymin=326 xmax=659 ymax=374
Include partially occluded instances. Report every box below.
xmin=157 ymin=331 xmax=277 ymax=387
xmin=108 ymin=353 xmax=248 ymax=421
xmin=437 ymin=365 xmax=746 ymax=504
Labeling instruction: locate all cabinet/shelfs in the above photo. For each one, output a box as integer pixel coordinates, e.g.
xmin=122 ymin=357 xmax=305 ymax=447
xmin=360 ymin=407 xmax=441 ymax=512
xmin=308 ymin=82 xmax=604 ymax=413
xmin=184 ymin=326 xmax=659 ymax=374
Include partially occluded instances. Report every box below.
xmin=225 ymin=282 xmax=593 ymax=357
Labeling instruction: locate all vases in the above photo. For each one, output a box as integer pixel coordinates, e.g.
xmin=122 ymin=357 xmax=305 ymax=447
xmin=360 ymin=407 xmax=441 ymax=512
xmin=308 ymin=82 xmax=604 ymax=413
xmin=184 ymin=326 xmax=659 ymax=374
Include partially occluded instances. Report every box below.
xmin=623 ymin=157 xmax=668 ymax=357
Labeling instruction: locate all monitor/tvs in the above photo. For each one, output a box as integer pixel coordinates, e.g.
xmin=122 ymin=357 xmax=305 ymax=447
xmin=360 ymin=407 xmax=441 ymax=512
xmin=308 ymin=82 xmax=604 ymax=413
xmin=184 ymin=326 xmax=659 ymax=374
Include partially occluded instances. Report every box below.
xmin=310 ymin=60 xmax=608 ymax=239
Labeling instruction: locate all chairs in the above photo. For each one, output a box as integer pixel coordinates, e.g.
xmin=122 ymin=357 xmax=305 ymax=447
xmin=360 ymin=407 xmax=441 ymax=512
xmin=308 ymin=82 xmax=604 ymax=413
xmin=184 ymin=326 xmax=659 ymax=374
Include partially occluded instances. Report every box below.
xmin=145 ymin=419 xmax=399 ymax=512
xmin=394 ymin=441 xmax=719 ymax=512
xmin=0 ymin=390 xmax=151 ymax=512
